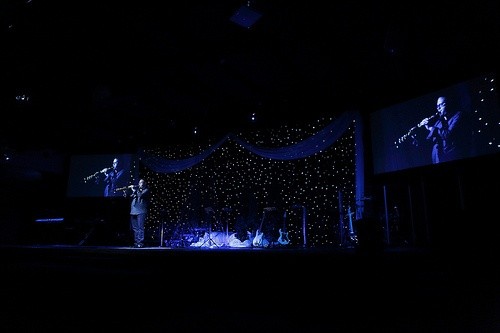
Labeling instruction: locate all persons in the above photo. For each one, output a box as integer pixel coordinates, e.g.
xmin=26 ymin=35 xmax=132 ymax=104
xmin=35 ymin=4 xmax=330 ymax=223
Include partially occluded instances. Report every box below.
xmin=129 ymin=179 xmax=150 ymax=248
xmin=102 ymin=157 xmax=128 ymax=197
xmin=409 ymin=93 xmax=466 ymax=165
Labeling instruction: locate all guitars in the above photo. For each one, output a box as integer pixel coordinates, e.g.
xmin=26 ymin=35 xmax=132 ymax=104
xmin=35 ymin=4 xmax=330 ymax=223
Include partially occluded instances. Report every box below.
xmin=277 ymin=211 xmax=291 ymax=246
xmin=252 ymin=212 xmax=265 ymax=247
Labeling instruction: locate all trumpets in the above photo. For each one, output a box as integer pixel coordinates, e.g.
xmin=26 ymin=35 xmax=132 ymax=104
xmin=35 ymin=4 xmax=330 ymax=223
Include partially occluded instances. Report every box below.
xmin=84 ymin=166 xmax=114 ymax=184
xmin=393 ymin=110 xmax=442 ymax=150
xmin=113 ymin=185 xmax=140 ymax=198
